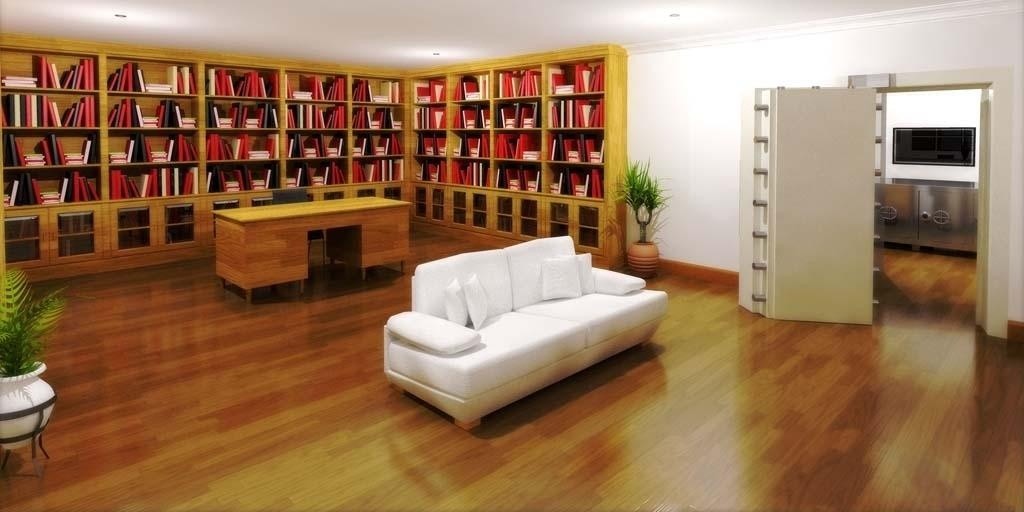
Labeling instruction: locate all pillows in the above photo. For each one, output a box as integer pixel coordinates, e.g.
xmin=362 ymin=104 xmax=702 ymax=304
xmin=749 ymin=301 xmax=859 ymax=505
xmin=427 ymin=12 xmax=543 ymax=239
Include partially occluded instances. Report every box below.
xmin=541 ymin=252 xmax=594 ymax=301
xmin=443 ymin=273 xmax=489 ymax=331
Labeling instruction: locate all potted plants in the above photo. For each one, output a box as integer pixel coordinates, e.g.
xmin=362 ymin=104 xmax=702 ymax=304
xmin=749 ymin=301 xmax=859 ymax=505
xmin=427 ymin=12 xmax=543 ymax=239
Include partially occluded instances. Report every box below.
xmin=608 ymin=153 xmax=676 ymax=280
xmin=1 ymin=267 xmax=70 ymax=453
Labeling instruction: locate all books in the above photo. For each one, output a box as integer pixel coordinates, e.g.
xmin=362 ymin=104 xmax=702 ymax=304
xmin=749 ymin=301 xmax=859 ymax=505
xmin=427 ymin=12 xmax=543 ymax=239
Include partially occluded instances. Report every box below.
xmin=288 ymin=75 xmax=346 ymax=186
xmin=206 ymin=68 xmax=279 ymax=192
xmin=352 ymin=79 xmax=404 ymax=183
xmin=107 ymin=62 xmax=198 ymax=200
xmin=1 ymin=56 xmax=99 ymax=208
xmin=415 ymin=74 xmax=490 ymax=188
xmin=496 ymin=69 xmax=542 ymax=193
xmin=547 ymin=61 xmax=604 ymax=198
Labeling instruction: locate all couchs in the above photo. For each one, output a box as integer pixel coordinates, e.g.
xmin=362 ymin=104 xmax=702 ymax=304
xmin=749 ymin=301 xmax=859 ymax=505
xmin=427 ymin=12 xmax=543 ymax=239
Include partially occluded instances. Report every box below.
xmin=382 ymin=235 xmax=671 ymax=432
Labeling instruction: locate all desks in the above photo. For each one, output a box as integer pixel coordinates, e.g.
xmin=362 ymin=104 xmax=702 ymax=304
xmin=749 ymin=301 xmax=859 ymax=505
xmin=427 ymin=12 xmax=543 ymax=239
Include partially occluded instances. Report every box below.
xmin=211 ymin=196 xmax=413 ymax=305
